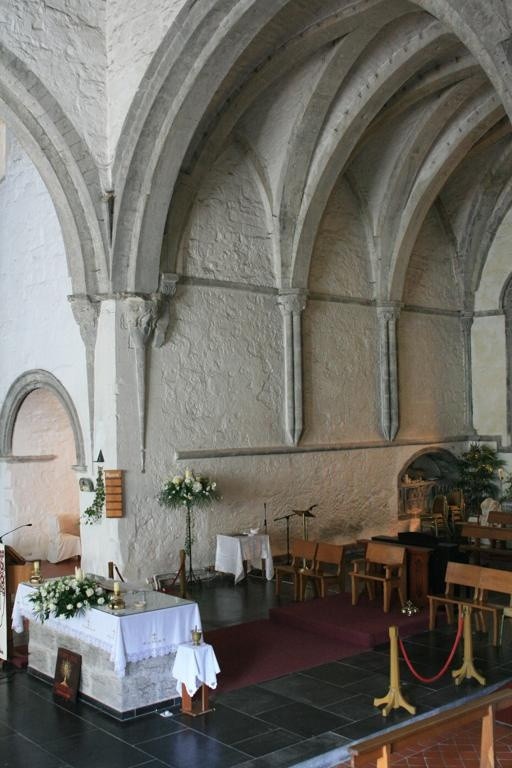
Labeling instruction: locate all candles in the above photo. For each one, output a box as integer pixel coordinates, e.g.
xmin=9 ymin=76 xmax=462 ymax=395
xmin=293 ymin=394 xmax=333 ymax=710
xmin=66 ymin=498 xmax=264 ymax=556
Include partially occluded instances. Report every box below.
xmin=113 ymin=582 xmax=120 ymax=592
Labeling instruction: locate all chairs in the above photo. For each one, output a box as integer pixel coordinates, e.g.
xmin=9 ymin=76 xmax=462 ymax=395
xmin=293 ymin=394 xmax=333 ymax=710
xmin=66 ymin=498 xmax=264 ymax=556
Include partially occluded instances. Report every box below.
xmin=275 ymin=540 xmax=319 ymax=600
xmin=446 ymin=488 xmax=465 ymax=534
xmin=299 ymin=542 xmax=345 ymax=601
xmin=420 ymin=495 xmax=452 ymax=537
xmin=348 ymin=543 xmax=407 ymax=614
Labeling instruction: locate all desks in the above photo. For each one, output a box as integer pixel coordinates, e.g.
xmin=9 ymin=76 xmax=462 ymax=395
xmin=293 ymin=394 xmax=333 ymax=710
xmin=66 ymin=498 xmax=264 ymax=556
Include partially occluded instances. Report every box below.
xmin=11 ymin=574 xmax=204 ymax=723
xmin=214 ymin=532 xmax=275 ymax=585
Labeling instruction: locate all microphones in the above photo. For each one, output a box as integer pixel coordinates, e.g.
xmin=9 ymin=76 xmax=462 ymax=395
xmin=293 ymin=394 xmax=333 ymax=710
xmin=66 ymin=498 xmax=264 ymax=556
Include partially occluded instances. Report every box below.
xmin=0 ymin=523 xmax=33 ymax=544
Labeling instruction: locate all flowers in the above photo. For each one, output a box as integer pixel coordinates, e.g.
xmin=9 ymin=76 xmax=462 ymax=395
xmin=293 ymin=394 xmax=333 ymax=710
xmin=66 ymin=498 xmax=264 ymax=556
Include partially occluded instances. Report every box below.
xmin=158 ymin=465 xmax=224 ymax=508
xmin=24 ymin=576 xmax=109 ymax=623
xmin=81 ymin=504 xmax=102 ymax=526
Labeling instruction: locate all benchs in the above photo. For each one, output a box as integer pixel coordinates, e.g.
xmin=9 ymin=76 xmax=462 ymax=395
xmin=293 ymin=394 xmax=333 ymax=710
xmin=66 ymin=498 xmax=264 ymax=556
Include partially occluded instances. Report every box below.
xmin=459 ymin=527 xmax=512 ymax=557
xmin=425 ymin=561 xmax=512 ymax=647
xmin=487 ymin=511 xmax=512 ymax=528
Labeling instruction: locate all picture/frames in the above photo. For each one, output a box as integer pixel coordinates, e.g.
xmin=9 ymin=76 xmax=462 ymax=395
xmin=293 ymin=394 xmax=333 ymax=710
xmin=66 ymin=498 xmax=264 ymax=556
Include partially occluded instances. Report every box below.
xmin=52 ymin=648 xmax=81 ymax=708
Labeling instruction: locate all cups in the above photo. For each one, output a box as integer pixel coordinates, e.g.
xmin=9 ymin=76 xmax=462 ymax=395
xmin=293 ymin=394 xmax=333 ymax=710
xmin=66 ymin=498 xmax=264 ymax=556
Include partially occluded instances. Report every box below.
xmin=249 ymin=528 xmax=260 ymax=534
xmin=132 ymin=591 xmax=147 ymax=608
